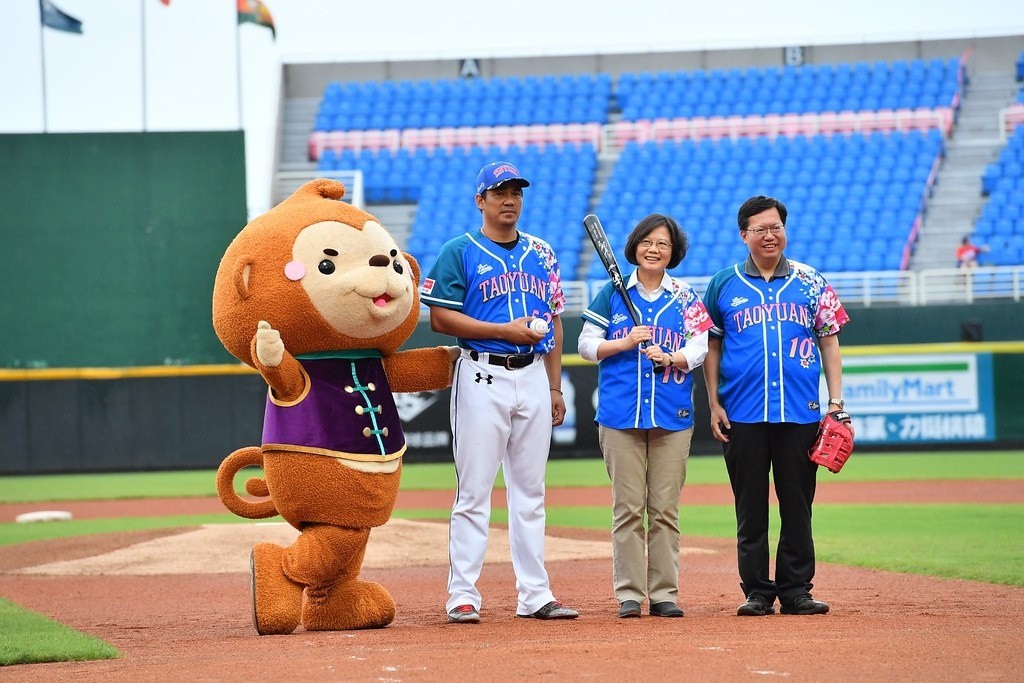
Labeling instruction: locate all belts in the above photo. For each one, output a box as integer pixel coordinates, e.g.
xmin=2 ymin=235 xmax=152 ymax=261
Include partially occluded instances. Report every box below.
xmin=470 ymin=350 xmax=534 ymax=370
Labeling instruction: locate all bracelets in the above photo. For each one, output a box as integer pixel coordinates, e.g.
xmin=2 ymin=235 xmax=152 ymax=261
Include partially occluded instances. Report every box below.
xmin=550 ymin=387 xmax=563 ymax=395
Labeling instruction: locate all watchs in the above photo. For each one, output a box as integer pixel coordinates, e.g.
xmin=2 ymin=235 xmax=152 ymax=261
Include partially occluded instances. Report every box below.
xmin=827 ymin=399 xmax=845 ymax=409
xmin=666 ymin=352 xmax=674 ymax=368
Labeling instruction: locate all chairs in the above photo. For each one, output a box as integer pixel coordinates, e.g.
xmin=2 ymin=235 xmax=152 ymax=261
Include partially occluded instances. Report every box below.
xmin=308 ymin=54 xmax=1024 ymax=316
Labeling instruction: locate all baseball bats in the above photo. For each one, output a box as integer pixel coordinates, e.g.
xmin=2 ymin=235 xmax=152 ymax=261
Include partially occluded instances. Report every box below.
xmin=583 ymin=214 xmax=666 ymax=374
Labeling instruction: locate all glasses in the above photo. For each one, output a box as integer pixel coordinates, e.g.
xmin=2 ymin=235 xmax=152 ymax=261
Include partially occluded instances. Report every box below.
xmin=638 ymin=236 xmax=672 ymax=250
xmin=744 ymin=223 xmax=784 ymax=237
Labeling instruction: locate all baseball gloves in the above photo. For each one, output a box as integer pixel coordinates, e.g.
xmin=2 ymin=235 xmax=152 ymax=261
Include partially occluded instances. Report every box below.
xmin=810 ymin=409 xmax=855 ymax=473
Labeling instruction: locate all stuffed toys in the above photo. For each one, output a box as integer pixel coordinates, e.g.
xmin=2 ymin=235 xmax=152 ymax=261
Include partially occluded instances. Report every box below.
xmin=211 ymin=177 xmax=461 ymax=635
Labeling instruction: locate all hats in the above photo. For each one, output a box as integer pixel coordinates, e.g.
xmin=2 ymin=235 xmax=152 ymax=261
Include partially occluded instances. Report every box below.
xmin=476 ymin=161 xmax=530 ymax=194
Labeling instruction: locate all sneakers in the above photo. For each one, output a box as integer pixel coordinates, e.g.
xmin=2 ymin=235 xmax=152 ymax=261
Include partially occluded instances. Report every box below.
xmin=517 ymin=601 xmax=578 ymax=619
xmin=619 ymin=600 xmax=641 ymax=616
xmin=448 ymin=605 xmax=480 ymax=622
xmin=737 ymin=592 xmax=775 ymax=615
xmin=649 ymin=602 xmax=683 ymax=617
xmin=779 ymin=596 xmax=829 ymax=615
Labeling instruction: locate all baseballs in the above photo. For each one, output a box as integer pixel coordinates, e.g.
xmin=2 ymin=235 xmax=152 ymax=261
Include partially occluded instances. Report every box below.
xmin=530 ymin=318 xmax=548 ymax=335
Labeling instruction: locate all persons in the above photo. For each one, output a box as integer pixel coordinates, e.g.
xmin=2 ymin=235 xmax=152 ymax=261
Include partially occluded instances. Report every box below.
xmin=701 ymin=196 xmax=855 ymax=616
xmin=577 ymin=214 xmax=714 ymax=618
xmin=958 ymin=237 xmax=990 ymax=269
xmin=419 ymin=161 xmax=579 ymax=622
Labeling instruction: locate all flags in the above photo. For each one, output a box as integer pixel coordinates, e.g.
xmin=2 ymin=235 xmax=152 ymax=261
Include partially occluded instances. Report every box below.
xmin=235 ymin=0 xmax=278 ymax=43
xmin=38 ymin=0 xmax=85 ymax=36
xmin=158 ymin=0 xmax=172 ymax=8
